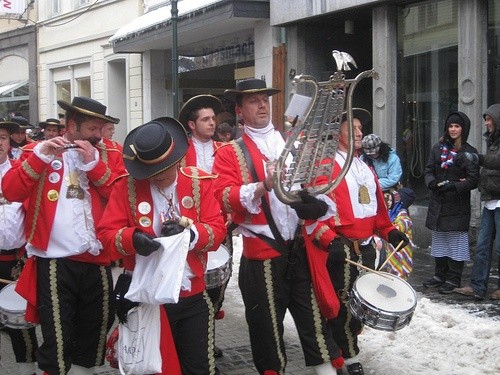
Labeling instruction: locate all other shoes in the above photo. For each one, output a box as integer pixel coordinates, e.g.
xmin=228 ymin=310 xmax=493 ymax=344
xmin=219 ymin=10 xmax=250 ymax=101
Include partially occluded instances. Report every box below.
xmin=423 ymin=278 xmax=444 ymax=289
xmin=346 ymin=361 xmax=364 ymax=375
xmin=489 ymin=289 xmax=500 ymax=300
xmin=439 ymin=282 xmax=461 ymax=295
xmin=452 ymin=286 xmax=483 ymax=301
xmin=214 ymin=345 xmax=223 ymax=358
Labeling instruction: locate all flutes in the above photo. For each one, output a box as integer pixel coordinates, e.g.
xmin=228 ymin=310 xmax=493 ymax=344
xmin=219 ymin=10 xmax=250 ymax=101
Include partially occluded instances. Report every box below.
xmin=53 ymin=137 xmax=102 ymax=150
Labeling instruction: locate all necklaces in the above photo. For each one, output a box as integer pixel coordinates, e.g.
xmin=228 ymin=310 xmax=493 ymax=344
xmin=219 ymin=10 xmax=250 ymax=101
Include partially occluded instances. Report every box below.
xmin=155 ymin=185 xmax=176 ymax=206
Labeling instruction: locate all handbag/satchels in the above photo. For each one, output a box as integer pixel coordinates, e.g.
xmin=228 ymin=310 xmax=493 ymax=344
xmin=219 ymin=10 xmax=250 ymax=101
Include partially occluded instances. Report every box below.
xmin=115 ymin=304 xmax=180 ymax=375
xmin=304 ymin=231 xmax=340 ymax=320
xmin=124 ymin=227 xmax=191 ymax=305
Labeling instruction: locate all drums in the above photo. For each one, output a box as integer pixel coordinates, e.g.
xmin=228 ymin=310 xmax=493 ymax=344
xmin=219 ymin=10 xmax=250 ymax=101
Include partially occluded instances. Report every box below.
xmin=202 ymin=241 xmax=232 ymax=289
xmin=348 ymin=271 xmax=419 ymax=333
xmin=0 ymin=279 xmax=40 ymax=330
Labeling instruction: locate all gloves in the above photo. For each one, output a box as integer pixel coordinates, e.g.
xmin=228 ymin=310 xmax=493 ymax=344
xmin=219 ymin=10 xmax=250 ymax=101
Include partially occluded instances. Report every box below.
xmin=388 ymin=228 xmax=409 ymax=252
xmin=429 ymin=180 xmax=455 ymax=192
xmin=161 ymin=219 xmax=195 ymax=245
xmin=133 ymin=229 xmax=162 ymax=256
xmin=290 ymin=193 xmax=330 ymax=220
xmin=328 ymin=239 xmax=347 ymax=264
xmin=453 ymin=151 xmax=479 ymax=169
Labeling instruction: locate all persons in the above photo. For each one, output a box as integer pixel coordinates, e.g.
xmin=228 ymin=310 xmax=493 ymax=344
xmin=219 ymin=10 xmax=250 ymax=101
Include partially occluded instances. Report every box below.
xmin=423 ymin=110 xmax=480 ymax=294
xmin=0 ymin=78 xmax=414 ymax=375
xmin=452 ymin=104 xmax=500 ymax=301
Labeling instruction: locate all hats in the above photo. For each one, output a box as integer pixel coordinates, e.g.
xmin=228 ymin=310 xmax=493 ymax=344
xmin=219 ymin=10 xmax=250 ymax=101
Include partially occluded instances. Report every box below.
xmin=362 ymin=134 xmax=384 ymax=156
xmin=38 ymin=118 xmax=65 ymax=130
xmin=0 ymin=112 xmax=19 ymax=135
xmin=326 ymin=106 xmax=371 ymax=138
xmin=179 ymin=93 xmax=224 ymax=130
xmin=123 ymin=116 xmax=190 ymax=181
xmin=445 ymin=114 xmax=463 ymax=128
xmin=224 ymin=79 xmax=281 ymax=102
xmin=10 ymin=115 xmax=35 ymax=130
xmin=57 ymin=95 xmax=118 ymax=124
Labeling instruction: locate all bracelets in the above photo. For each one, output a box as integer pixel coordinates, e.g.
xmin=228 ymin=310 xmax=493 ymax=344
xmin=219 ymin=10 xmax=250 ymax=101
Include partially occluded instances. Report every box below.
xmin=264 ymin=179 xmax=272 ymax=193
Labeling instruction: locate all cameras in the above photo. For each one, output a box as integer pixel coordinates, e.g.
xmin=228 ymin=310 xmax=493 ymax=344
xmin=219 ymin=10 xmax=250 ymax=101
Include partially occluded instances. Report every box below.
xmin=438 ymin=180 xmax=449 ymax=187
